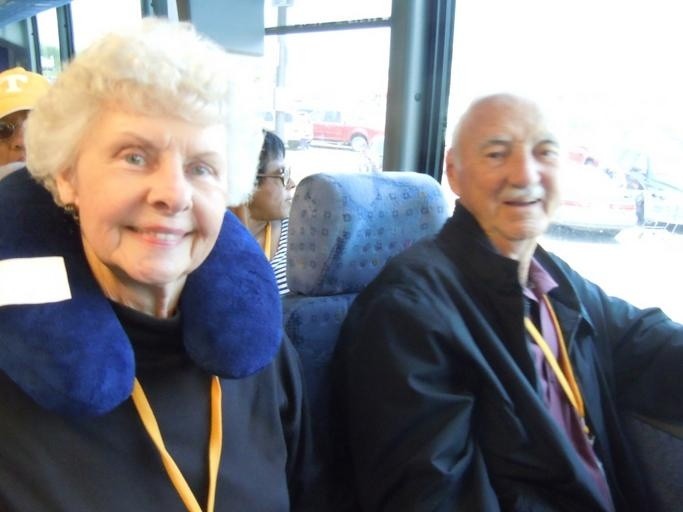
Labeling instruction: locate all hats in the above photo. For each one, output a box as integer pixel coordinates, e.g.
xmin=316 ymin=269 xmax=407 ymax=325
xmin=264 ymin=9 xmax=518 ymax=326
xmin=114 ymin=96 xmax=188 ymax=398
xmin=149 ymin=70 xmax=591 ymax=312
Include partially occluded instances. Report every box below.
xmin=1 ymin=66 xmax=52 ymax=119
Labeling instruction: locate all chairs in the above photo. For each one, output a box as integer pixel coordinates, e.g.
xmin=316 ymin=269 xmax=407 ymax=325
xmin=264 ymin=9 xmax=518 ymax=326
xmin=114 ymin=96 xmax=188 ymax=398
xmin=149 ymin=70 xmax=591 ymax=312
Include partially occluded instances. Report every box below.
xmin=276 ymin=167 xmax=453 ymax=511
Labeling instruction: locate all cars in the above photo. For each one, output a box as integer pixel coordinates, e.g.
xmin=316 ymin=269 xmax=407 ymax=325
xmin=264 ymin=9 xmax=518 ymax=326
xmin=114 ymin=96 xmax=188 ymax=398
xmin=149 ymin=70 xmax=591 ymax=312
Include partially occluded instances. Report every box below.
xmin=553 ymin=153 xmax=679 ymax=233
xmin=268 ymin=107 xmax=378 ymax=153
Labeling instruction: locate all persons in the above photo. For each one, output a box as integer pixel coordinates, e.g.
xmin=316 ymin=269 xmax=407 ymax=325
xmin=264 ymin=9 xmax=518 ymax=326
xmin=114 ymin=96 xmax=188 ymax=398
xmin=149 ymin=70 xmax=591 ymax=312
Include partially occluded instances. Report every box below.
xmin=1 ymin=11 xmax=334 ymax=512
xmin=226 ymin=127 xmax=301 ymax=294
xmin=333 ymin=90 xmax=683 ymax=512
xmin=0 ymin=62 xmax=57 ymax=174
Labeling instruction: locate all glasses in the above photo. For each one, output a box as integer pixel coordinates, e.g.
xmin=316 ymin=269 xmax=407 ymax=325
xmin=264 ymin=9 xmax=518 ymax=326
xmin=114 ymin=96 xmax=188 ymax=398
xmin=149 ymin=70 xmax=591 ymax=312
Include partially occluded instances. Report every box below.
xmin=257 ymin=168 xmax=292 ymax=186
